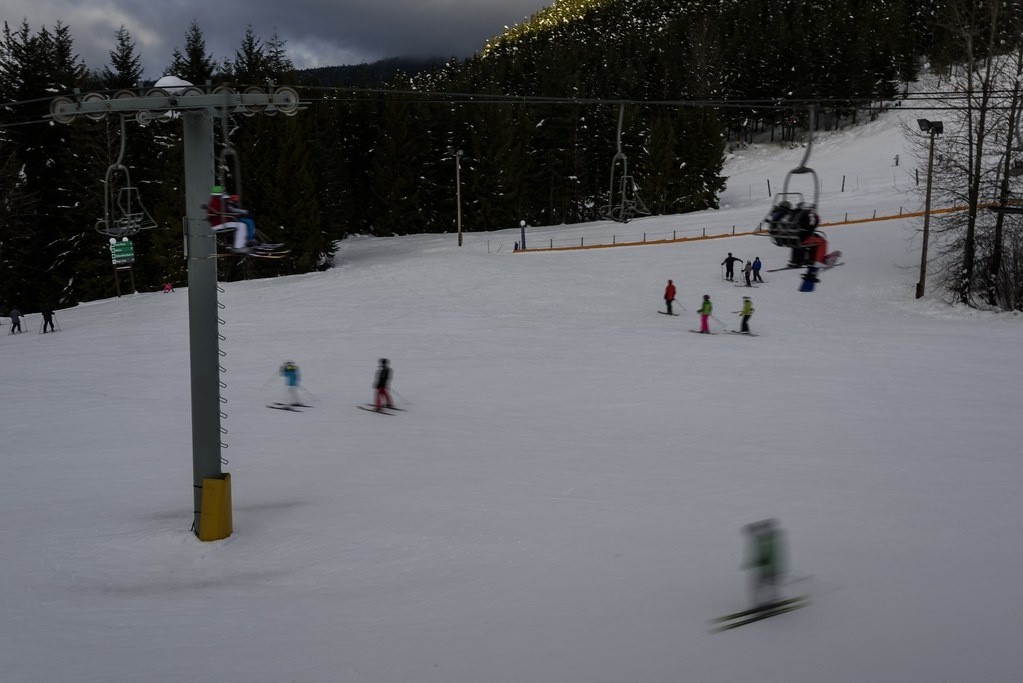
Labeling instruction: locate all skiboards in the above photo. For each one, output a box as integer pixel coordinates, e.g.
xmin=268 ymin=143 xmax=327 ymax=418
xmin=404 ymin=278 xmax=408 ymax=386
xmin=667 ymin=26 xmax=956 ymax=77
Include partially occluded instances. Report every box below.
xmin=265 ymin=402 xmax=316 ymax=413
xmin=688 ymin=330 xmax=720 ymax=335
xmin=702 ymin=594 xmax=814 ymax=634
xmin=39 ymin=329 xmax=62 ymax=334
xmin=728 ymin=329 xmax=759 ymax=337
xmin=657 ymin=310 xmax=680 ymax=316
xmin=733 ymin=285 xmax=759 ymax=288
xmin=724 ymin=279 xmax=738 ymax=283
xmin=743 ymin=280 xmax=769 ymax=283
xmin=354 ymin=403 xmax=408 ymax=417
xmin=8 ymin=330 xmax=28 ymax=336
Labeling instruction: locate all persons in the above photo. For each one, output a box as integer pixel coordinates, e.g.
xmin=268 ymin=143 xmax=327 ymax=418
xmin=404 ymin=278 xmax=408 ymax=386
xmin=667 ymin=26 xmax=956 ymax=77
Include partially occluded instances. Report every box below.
xmin=664 ymin=280 xmax=677 ymax=315
xmin=696 ymin=294 xmax=713 ymax=333
xmin=369 ymin=358 xmax=396 ymax=412
xmin=163 ymin=281 xmax=174 ymax=293
xmin=751 ymin=257 xmax=763 ymax=282
xmin=9 ymin=307 xmax=25 ymax=334
xmin=738 ymin=297 xmax=755 ymax=332
xmin=764 ymin=197 xmax=830 ymax=269
xmin=737 ymin=517 xmax=795 ymax=617
xmin=41 ymin=302 xmax=56 ymax=333
xmin=279 ymin=360 xmax=304 ymax=411
xmin=741 ymin=260 xmax=752 ymax=287
xmin=893 ymin=154 xmax=899 ymax=166
xmin=207 ymin=185 xmax=257 ymax=254
xmin=721 ymin=253 xmax=743 ymax=282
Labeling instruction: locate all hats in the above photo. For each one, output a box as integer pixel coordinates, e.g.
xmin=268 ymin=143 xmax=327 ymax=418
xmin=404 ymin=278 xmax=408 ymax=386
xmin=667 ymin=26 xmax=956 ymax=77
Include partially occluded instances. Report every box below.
xmin=222 ymin=192 xmax=231 ymax=199
xmin=210 ymin=186 xmax=224 ymax=195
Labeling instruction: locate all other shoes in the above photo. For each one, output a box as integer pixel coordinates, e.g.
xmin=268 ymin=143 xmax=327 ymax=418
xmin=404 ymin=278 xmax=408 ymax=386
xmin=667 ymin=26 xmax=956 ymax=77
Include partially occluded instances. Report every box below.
xmin=248 ymin=239 xmax=261 ymax=246
xmin=232 ymin=246 xmax=251 ymax=253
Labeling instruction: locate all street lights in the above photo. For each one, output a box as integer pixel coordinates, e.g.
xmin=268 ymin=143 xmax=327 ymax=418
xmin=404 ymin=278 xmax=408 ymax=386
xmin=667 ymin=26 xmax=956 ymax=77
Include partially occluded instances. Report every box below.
xmin=449 ymin=148 xmax=465 ymax=246
xmin=916 ymin=117 xmax=944 ymax=300
